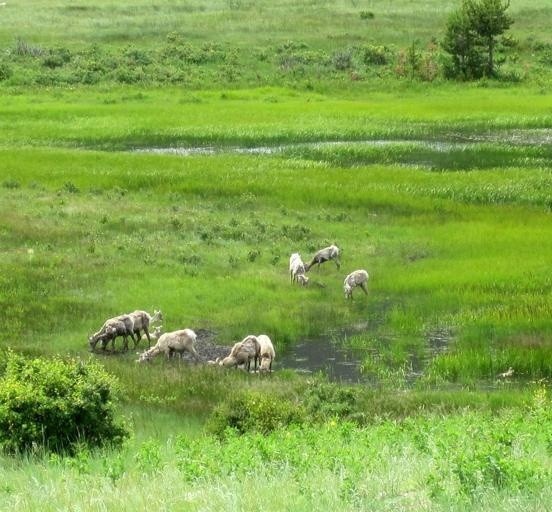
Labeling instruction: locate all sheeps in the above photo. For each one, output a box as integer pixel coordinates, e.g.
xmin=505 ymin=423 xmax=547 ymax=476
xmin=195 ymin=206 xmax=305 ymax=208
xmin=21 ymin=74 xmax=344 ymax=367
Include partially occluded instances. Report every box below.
xmin=207 ymin=357 xmax=220 ymax=365
xmin=136 ymin=329 xmax=199 ymax=365
xmin=344 ymin=270 xmax=369 ymax=299
xmin=304 ymin=245 xmax=340 ymax=274
xmin=88 ymin=309 xmax=163 ymax=354
xmin=290 ymin=253 xmax=309 ymax=289
xmin=218 ymin=335 xmax=275 ymax=373
